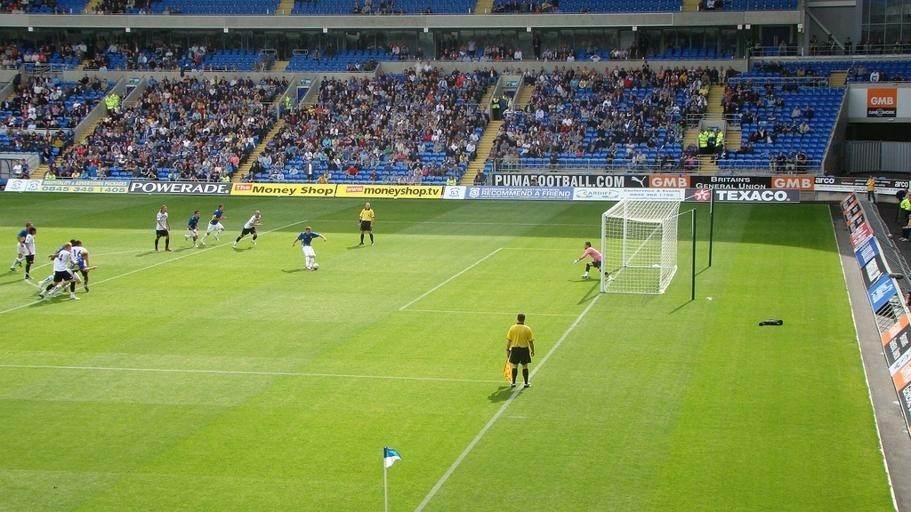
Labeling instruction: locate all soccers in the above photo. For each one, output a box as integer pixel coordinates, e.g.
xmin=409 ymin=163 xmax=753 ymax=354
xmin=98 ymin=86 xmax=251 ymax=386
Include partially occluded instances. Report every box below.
xmin=313 ymin=263 xmax=319 ymax=269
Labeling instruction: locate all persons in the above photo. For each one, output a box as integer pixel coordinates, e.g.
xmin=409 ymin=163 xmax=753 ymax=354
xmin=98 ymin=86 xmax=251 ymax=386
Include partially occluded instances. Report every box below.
xmin=11 ymin=222 xmax=92 ymax=302
xmin=292 ymin=226 xmax=327 ymax=270
xmin=506 ymin=312 xmax=535 ymax=388
xmin=866 ymin=175 xmax=911 ymax=241
xmin=151 ymin=203 xmax=260 ymax=254
xmin=356 ymin=202 xmax=374 ymax=247
xmin=574 ymin=242 xmax=615 ymax=282
xmin=0 ymin=2 xmax=902 ymax=178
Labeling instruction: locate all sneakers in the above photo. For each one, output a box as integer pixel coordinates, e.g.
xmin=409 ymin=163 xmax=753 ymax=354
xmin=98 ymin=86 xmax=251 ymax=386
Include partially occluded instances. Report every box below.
xmin=181 ymin=234 xmax=257 ymax=248
xmin=524 ymin=384 xmax=529 ymax=387
xmin=512 ymin=384 xmax=516 ymax=387
xmin=11 ymin=267 xmax=88 ymax=300
xmin=581 ymin=275 xmax=590 ymax=280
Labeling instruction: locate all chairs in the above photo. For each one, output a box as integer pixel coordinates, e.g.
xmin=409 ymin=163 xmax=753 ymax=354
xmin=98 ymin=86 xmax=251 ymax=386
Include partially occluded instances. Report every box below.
xmin=0 ymin=0 xmax=911 ymax=175
